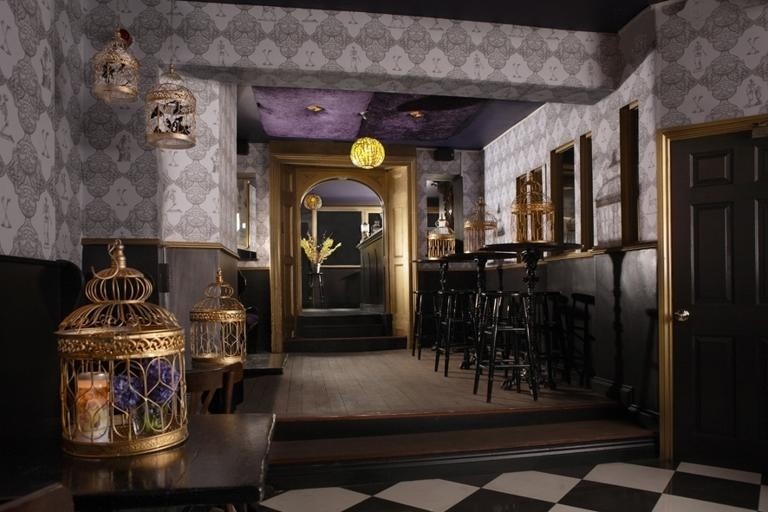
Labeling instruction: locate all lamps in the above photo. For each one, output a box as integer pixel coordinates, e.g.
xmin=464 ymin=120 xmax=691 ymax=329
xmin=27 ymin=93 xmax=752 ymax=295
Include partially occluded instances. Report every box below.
xmin=349 ymin=118 xmax=386 ymax=170
xmin=302 ymin=188 xmax=323 ymax=211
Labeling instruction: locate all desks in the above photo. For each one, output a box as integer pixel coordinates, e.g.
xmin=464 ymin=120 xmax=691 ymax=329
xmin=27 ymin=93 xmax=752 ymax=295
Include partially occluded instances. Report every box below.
xmin=59 ymin=409 xmax=277 ymax=509
xmin=411 ymin=239 xmax=584 ymax=386
xmin=240 ymin=349 xmax=291 ymax=378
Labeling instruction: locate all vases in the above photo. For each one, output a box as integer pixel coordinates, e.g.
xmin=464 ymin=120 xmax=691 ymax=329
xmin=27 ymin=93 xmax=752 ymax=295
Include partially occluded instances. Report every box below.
xmin=130 ymin=401 xmax=165 ymax=436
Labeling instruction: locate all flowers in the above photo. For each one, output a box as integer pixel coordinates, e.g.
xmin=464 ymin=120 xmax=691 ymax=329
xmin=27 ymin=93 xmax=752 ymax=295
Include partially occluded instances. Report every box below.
xmin=110 ymin=361 xmax=181 ymax=408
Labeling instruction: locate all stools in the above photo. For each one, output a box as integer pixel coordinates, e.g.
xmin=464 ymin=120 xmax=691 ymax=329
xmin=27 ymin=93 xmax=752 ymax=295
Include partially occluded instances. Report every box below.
xmin=410 ymin=285 xmax=598 ymax=402
